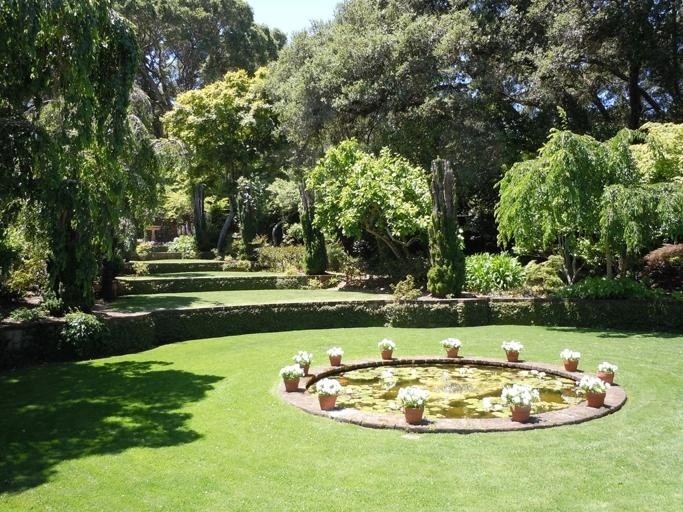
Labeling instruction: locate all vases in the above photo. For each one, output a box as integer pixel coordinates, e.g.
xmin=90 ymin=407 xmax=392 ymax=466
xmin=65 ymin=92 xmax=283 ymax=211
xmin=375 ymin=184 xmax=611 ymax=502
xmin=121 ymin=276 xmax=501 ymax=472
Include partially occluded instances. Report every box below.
xmin=381 ymin=349 xmax=393 ymax=359
xmin=283 ymin=365 xmax=308 ymax=391
xmin=511 ymin=404 xmax=531 ymax=422
xmin=505 ymin=350 xmax=519 ymax=362
xmin=445 ymin=346 xmax=459 ymax=358
xmin=584 ymin=371 xmax=613 ymax=407
xmin=405 ymin=406 xmax=424 ymax=424
xmin=563 ymin=359 xmax=577 ymax=371
xmin=328 ymin=355 xmax=342 ymax=367
xmin=318 ymin=394 xmax=339 ymax=410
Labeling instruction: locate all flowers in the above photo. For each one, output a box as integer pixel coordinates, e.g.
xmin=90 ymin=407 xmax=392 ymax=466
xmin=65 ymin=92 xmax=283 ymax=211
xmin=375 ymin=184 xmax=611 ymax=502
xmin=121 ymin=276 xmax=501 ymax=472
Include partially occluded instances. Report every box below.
xmin=579 ymin=360 xmax=617 ymax=393
xmin=315 ymin=376 xmax=341 ymax=395
xmin=559 ymin=349 xmax=580 ymax=361
xmin=378 ymin=339 xmax=394 ymax=349
xmin=278 ymin=350 xmax=314 ymax=381
xmin=501 ymin=383 xmax=540 ymax=406
xmin=327 ymin=347 xmax=342 ymax=356
xmin=396 ymin=385 xmax=429 ymax=406
xmin=442 ymin=337 xmax=462 ymax=347
xmin=502 ymin=341 xmax=523 ymax=352
